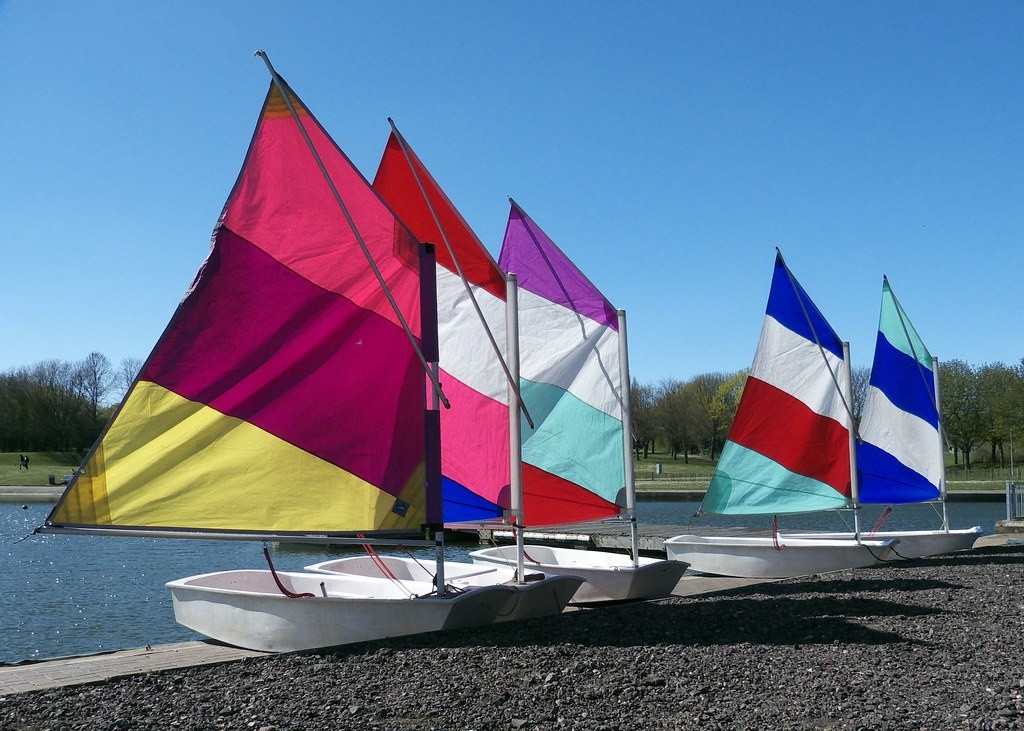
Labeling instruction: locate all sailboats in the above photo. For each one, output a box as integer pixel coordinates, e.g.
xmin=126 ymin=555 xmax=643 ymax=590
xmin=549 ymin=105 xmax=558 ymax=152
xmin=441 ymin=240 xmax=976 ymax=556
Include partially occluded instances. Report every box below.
xmin=35 ymin=48 xmax=515 ymax=653
xmin=303 ymin=117 xmax=584 ymax=623
xmin=773 ymin=275 xmax=985 ymax=557
xmin=663 ymin=246 xmax=898 ymax=578
xmin=467 ymin=195 xmax=690 ymax=603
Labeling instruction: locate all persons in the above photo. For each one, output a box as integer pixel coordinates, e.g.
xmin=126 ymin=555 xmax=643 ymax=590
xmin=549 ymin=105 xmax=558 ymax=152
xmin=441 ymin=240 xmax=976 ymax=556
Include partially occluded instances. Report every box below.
xmin=19 ymin=454 xmax=29 ymax=471
xmin=71 ymin=469 xmax=76 ymax=477
